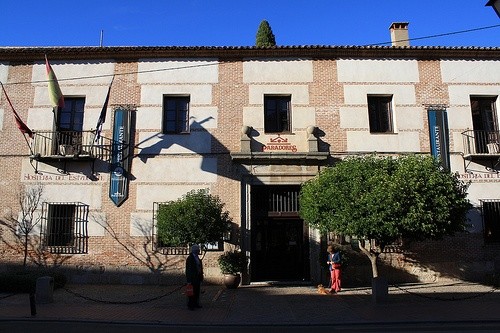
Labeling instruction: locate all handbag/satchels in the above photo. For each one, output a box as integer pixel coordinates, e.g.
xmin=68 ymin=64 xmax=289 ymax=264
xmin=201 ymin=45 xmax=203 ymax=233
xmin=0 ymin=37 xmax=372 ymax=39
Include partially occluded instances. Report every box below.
xmin=184 ymin=284 xmax=193 ymax=296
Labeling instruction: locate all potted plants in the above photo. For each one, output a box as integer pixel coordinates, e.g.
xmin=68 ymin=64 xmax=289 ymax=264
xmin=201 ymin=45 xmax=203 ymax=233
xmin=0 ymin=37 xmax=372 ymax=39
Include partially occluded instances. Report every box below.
xmin=218 ymin=250 xmax=246 ymax=290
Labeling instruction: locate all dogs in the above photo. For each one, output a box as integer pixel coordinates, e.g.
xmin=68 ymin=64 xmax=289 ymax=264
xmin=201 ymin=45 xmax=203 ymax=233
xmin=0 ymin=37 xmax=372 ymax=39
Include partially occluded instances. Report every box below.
xmin=317 ymin=284 xmax=329 ymax=295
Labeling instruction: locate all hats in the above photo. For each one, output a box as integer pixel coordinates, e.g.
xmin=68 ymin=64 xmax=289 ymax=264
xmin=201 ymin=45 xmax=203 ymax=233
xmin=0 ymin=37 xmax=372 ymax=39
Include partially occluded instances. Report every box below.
xmin=190 ymin=245 xmax=199 ymax=253
xmin=327 ymin=246 xmax=333 ymax=252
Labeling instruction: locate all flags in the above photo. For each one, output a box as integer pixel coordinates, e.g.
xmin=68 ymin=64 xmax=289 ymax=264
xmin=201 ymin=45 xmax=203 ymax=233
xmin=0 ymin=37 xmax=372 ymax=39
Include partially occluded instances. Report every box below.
xmin=4 ymin=91 xmax=34 ymax=139
xmin=94 ymin=87 xmax=111 ymax=142
xmin=46 ymin=59 xmax=65 ymax=108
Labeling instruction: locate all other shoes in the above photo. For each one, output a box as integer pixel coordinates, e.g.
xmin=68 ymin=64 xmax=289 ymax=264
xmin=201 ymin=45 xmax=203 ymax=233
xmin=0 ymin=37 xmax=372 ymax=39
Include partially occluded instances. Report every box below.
xmin=188 ymin=306 xmax=202 ymax=311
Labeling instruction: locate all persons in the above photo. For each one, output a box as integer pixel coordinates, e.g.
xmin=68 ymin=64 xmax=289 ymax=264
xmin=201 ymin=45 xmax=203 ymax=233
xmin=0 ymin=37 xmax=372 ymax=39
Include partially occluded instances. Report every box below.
xmin=326 ymin=245 xmax=343 ymax=292
xmin=185 ymin=244 xmax=204 ymax=310
